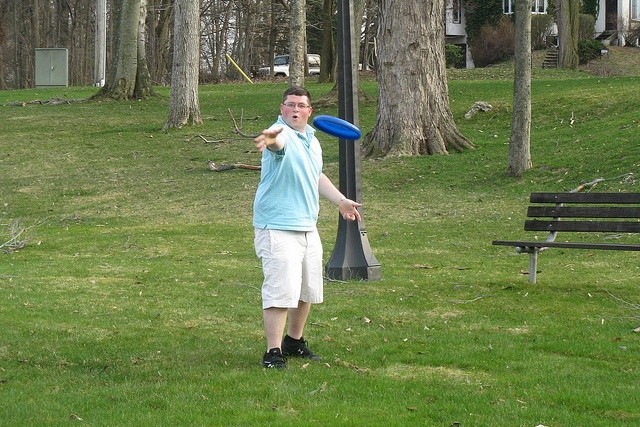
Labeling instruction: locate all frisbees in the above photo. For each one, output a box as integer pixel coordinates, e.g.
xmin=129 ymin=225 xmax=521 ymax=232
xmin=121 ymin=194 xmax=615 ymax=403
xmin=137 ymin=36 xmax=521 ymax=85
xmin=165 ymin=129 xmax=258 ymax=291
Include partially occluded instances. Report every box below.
xmin=312 ymin=115 xmax=362 ymax=139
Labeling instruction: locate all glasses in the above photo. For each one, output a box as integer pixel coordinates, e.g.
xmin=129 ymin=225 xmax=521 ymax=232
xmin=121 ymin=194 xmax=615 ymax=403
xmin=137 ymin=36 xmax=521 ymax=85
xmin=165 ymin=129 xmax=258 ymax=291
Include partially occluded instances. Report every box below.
xmin=282 ymin=101 xmax=310 ymax=108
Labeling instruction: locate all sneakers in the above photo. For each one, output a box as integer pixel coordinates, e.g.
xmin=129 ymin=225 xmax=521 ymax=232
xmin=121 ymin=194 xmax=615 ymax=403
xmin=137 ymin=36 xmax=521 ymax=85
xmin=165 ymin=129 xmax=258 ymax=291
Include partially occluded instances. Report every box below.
xmin=281 ymin=336 xmax=322 ymax=359
xmin=262 ymin=347 xmax=290 ymax=369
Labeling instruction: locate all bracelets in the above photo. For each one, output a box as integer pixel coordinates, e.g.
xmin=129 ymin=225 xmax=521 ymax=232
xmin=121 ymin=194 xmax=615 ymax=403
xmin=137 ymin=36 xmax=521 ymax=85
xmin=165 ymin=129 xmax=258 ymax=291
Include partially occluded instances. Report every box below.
xmin=336 ymin=197 xmax=346 ymax=206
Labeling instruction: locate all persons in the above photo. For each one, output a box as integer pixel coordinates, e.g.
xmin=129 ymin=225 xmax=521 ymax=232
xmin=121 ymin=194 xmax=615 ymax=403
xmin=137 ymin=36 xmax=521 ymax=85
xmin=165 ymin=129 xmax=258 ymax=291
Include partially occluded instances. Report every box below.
xmin=250 ymin=85 xmax=363 ymax=370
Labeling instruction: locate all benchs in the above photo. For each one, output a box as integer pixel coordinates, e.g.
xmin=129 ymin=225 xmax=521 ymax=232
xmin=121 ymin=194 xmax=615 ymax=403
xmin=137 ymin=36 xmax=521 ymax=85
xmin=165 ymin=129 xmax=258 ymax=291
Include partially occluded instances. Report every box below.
xmin=542 ymin=46 xmax=559 ymax=68
xmin=490 ymin=191 xmax=639 ymax=284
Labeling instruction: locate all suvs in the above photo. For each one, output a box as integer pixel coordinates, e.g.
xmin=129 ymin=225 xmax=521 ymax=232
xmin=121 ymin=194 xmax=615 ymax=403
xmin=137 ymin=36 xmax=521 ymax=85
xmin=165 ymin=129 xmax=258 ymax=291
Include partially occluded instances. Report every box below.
xmin=257 ymin=54 xmax=331 ymax=80
